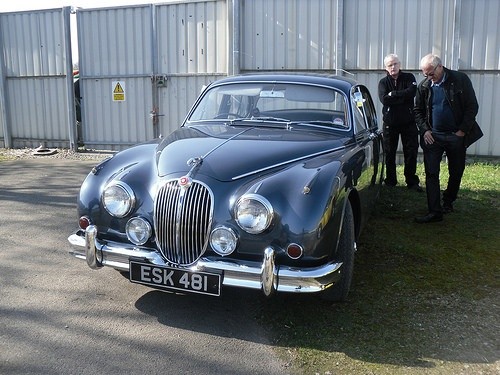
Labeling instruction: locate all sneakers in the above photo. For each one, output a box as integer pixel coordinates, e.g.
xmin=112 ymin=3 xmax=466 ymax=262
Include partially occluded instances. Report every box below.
xmin=407 ymin=183 xmax=423 ymax=193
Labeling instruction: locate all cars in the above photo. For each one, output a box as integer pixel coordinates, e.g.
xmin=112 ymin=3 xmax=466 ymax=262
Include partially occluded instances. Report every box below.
xmin=66 ymin=73 xmax=385 ymax=304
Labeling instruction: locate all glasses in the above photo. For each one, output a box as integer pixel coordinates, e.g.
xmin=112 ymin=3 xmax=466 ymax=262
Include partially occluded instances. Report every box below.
xmin=423 ymin=64 xmax=438 ymax=78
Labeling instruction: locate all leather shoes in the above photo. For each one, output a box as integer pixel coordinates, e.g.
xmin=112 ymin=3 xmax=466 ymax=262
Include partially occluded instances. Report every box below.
xmin=441 ymin=201 xmax=455 ymax=214
xmin=413 ymin=210 xmax=442 ymax=223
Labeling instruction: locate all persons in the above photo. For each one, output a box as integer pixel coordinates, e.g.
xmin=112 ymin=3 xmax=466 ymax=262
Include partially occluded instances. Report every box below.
xmin=414 ymin=54 xmax=484 ymax=223
xmin=378 ymin=54 xmax=423 ymax=192
